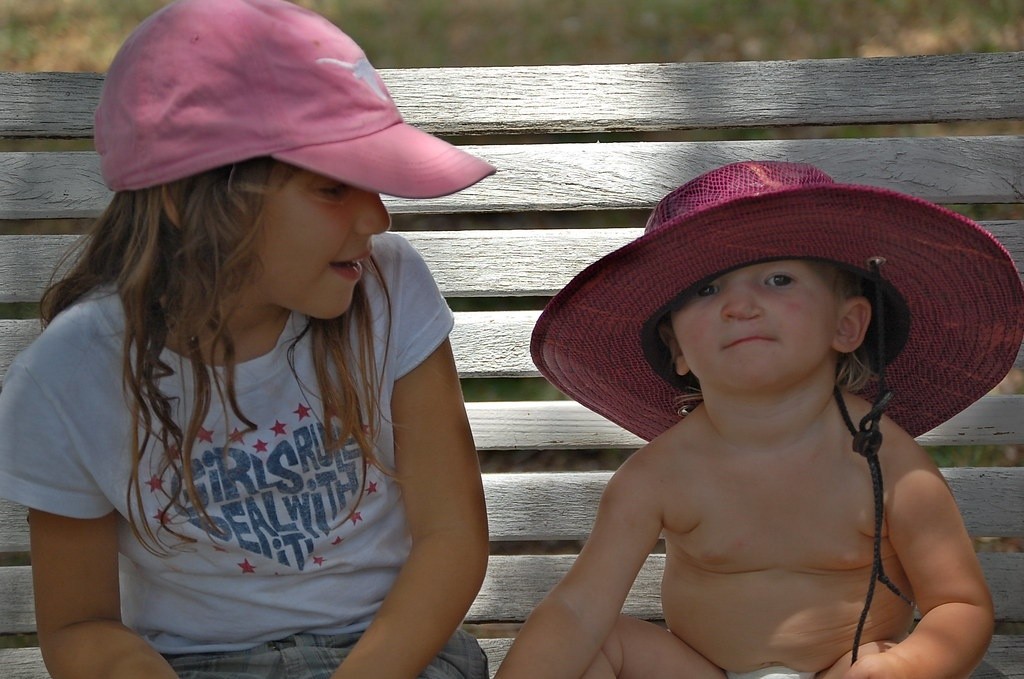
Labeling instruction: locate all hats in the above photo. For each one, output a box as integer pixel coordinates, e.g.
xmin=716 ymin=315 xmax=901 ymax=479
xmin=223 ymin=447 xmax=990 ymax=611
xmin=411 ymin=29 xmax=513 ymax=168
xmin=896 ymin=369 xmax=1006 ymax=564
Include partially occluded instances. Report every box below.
xmin=95 ymin=1 xmax=496 ymax=199
xmin=530 ymin=160 xmax=1024 ymax=442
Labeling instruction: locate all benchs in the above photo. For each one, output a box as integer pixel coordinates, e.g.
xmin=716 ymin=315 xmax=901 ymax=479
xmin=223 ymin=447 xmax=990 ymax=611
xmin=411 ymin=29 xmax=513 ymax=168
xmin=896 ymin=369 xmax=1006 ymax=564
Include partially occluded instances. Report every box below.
xmin=0 ymin=72 xmax=1024 ymax=679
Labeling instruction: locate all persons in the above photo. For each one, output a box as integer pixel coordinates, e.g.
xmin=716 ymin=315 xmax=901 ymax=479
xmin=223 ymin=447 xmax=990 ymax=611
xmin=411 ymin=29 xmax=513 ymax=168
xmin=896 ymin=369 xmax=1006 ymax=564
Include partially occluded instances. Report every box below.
xmin=0 ymin=1 xmax=501 ymax=678
xmin=494 ymin=159 xmax=1023 ymax=679
